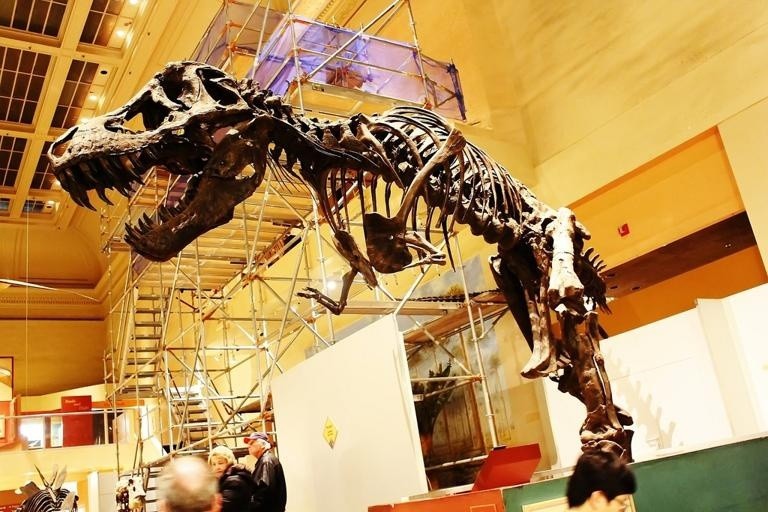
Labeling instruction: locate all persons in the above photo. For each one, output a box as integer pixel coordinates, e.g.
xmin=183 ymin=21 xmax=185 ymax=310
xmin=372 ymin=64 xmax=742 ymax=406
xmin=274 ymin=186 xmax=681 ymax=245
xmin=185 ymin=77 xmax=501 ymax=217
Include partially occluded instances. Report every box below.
xmin=156 ymin=454 xmax=224 ymax=511
xmin=117 ymin=478 xmax=135 ymax=512
xmin=206 ymin=444 xmax=256 ymax=512
xmin=561 ymin=451 xmax=636 ymax=512
xmin=244 ymin=430 xmax=288 ymax=512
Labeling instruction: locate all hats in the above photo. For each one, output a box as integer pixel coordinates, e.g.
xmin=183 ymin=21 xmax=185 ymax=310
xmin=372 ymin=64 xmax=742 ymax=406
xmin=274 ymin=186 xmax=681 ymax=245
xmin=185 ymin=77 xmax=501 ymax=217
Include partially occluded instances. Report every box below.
xmin=244 ymin=431 xmax=270 ymax=444
